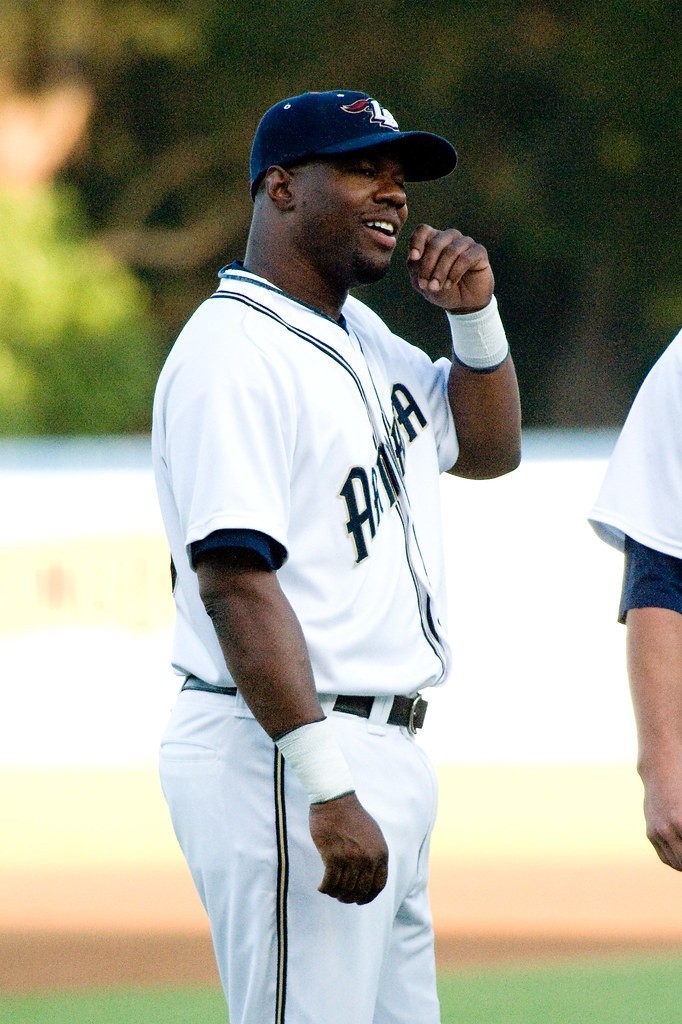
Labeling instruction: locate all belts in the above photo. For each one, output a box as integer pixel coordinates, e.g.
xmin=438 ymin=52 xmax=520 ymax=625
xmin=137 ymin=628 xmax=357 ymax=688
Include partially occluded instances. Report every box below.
xmin=181 ymin=666 xmax=429 ymax=731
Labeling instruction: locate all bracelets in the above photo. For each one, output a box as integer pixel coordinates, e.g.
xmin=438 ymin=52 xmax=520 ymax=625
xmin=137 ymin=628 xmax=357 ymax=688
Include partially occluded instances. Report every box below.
xmin=273 ymin=716 xmax=353 ymax=802
xmin=445 ymin=295 xmax=509 ymax=370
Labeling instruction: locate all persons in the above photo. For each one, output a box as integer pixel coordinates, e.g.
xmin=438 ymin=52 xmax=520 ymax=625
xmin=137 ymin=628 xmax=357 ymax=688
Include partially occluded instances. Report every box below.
xmin=587 ymin=324 xmax=682 ymax=871
xmin=149 ymin=92 xmax=520 ymax=1024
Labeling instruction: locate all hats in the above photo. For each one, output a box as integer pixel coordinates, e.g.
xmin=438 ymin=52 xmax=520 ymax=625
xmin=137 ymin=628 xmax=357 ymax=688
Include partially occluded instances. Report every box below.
xmin=250 ymin=86 xmax=460 ymax=201
